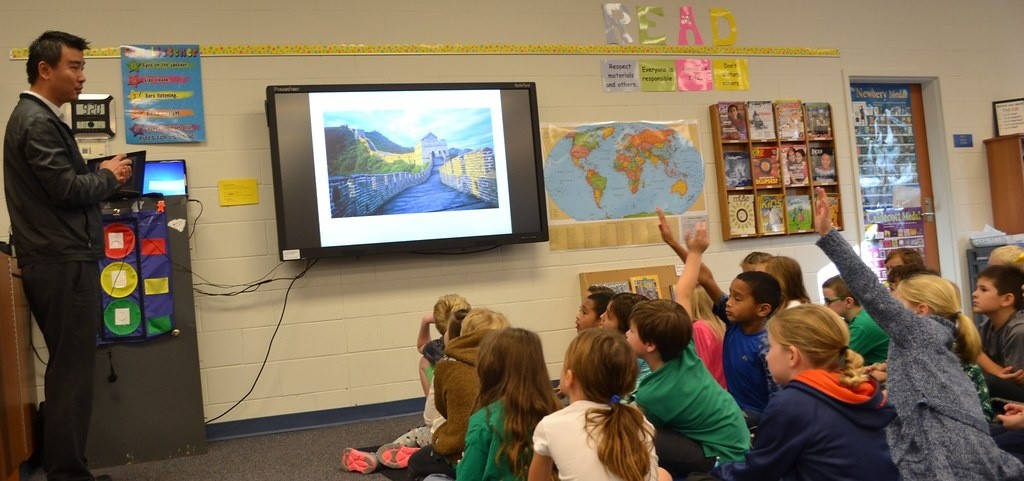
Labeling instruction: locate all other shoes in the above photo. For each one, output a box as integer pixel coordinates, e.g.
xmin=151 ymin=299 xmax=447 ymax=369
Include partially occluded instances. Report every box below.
xmin=93 ymin=474 xmax=112 ymax=481
xmin=376 ymin=443 xmax=421 ymax=469
xmin=341 ymin=444 xmax=383 ymax=475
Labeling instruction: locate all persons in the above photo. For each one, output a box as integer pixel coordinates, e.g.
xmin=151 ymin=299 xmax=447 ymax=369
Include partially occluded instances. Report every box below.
xmin=341 ymin=294 xmax=673 ymax=481
xmin=795 ymin=149 xmax=808 ymax=184
xmin=656 ymin=187 xmax=1024 ymax=481
xmin=787 ymin=147 xmax=795 ymax=184
xmin=728 ymin=105 xmax=745 ymax=132
xmin=3 ymin=31 xmax=132 ymax=481
xmin=814 ymin=153 xmax=836 ymax=182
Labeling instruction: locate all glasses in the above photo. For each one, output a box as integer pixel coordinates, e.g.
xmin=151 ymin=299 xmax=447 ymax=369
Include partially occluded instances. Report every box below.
xmin=824 ymin=297 xmax=843 ymax=306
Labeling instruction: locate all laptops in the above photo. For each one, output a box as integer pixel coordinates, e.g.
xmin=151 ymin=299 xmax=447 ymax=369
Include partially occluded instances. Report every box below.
xmin=143 ymin=159 xmax=189 ymax=202
xmin=86 ymin=150 xmax=147 ymax=200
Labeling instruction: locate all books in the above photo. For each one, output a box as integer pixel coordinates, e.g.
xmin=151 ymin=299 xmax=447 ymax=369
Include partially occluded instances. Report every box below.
xmin=717 ymin=100 xmax=841 ymax=235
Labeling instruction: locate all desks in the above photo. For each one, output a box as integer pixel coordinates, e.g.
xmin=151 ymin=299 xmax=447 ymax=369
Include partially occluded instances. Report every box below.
xmin=965 ymin=243 xmax=1024 ymax=326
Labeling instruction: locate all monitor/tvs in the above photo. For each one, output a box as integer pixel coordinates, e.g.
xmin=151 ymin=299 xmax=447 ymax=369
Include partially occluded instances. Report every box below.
xmin=264 ymin=81 xmax=550 ymax=263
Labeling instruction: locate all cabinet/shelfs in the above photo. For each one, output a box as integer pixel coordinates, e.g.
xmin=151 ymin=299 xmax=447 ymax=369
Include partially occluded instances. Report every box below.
xmin=983 ymin=133 xmax=1024 ymax=235
xmin=707 ymin=102 xmax=844 ymax=242
xmin=0 ymin=253 xmax=39 ymax=481
